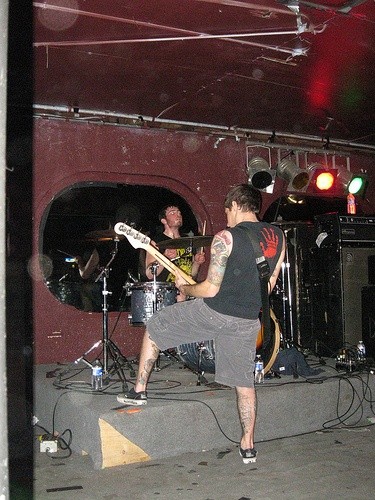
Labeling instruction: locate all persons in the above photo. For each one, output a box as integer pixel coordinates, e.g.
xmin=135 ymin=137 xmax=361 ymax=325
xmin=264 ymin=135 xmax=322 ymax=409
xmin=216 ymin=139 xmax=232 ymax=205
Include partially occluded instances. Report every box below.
xmin=145 ymin=203 xmax=205 ymax=302
xmin=117 ymin=184 xmax=286 ymax=464
xmin=76 ymin=221 xmax=128 ymax=311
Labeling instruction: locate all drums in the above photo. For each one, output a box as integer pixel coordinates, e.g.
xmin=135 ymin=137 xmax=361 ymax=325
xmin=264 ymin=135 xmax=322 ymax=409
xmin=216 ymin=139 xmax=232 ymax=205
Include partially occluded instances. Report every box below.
xmin=175 ymin=339 xmax=216 ymax=374
xmin=122 ymin=281 xmax=178 ymax=327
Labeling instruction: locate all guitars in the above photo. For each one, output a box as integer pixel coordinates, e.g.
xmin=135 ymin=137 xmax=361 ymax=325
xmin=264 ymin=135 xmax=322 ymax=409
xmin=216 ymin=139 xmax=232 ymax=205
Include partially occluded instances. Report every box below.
xmin=115 ymin=222 xmax=281 ymax=376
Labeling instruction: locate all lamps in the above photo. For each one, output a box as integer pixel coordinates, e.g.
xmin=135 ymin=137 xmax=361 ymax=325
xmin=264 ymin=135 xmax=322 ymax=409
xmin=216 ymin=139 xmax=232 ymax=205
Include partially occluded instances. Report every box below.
xmin=332 ymin=164 xmax=370 ymax=196
xmin=245 ymin=146 xmax=273 ymax=189
xmin=306 ymin=162 xmax=339 ymax=194
xmin=273 ymin=150 xmax=307 ymax=190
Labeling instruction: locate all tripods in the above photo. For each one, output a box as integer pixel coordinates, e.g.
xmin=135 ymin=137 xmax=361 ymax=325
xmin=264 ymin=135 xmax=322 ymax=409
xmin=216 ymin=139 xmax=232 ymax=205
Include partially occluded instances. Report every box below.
xmin=54 ymin=241 xmax=134 ymax=393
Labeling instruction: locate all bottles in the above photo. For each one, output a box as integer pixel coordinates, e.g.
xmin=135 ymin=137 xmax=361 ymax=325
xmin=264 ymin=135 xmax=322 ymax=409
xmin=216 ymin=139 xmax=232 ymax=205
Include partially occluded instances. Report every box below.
xmin=91 ymin=358 xmax=103 ymax=390
xmin=357 ymin=341 xmax=365 ymax=360
xmin=347 ymin=193 xmax=356 ymax=214
xmin=254 ymin=354 xmax=264 ymax=383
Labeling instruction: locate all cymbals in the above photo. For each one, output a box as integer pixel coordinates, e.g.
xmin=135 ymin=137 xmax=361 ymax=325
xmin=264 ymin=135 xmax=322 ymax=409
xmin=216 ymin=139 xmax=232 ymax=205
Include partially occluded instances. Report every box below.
xmin=81 ymin=230 xmax=125 ymax=241
xmin=155 ymin=235 xmax=213 ymax=249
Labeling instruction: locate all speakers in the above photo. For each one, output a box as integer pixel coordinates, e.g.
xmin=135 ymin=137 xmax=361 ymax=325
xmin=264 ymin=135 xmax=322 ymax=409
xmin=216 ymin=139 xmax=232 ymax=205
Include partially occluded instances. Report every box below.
xmin=310 ymin=247 xmax=375 ymax=358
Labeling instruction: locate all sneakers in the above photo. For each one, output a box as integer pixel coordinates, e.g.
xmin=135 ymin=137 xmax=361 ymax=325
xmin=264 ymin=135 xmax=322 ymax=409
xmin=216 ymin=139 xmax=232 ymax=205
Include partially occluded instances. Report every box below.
xmin=238 ymin=446 xmax=258 ymax=464
xmin=117 ymin=387 xmax=147 ymax=406
